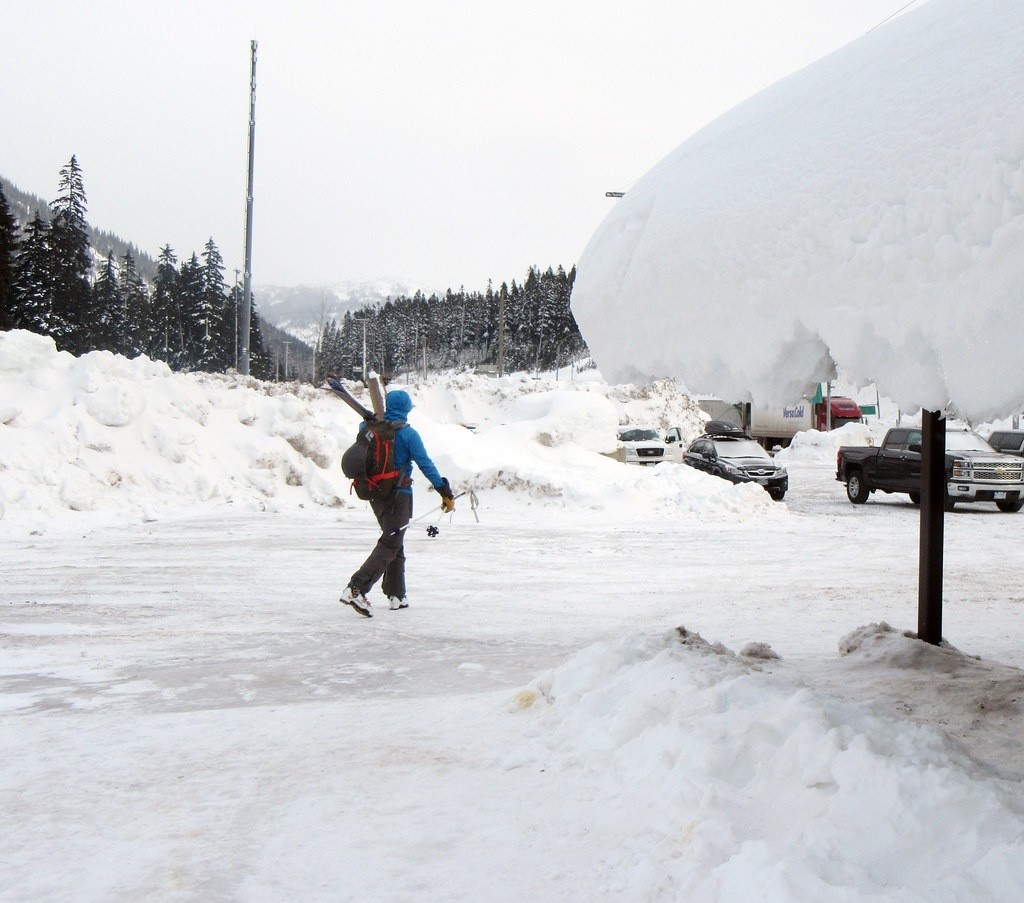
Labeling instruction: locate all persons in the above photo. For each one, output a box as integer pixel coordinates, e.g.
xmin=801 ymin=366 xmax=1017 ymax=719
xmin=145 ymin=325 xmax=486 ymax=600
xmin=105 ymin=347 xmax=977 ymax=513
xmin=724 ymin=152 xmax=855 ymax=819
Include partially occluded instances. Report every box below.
xmin=339 ymin=390 xmax=455 ymax=617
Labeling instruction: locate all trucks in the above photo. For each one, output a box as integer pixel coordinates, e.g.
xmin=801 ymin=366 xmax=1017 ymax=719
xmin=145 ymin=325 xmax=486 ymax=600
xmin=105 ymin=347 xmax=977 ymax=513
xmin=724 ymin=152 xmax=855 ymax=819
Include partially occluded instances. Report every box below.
xmin=696 ymin=380 xmax=864 ymax=451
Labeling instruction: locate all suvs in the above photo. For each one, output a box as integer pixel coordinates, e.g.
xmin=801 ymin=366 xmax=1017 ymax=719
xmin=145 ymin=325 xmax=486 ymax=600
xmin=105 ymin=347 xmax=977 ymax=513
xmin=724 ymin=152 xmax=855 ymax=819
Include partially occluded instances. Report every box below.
xmin=682 ymin=420 xmax=790 ymax=502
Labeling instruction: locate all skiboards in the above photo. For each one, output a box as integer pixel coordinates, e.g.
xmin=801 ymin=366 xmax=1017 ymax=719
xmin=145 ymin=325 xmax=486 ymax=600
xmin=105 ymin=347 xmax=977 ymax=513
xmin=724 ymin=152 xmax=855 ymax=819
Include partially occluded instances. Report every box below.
xmin=319 ymin=373 xmax=378 ymax=423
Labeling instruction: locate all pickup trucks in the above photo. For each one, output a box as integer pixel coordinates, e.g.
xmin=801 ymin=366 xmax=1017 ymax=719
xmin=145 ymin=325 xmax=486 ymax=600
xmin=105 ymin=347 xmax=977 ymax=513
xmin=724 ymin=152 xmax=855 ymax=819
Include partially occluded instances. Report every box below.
xmin=834 ymin=426 xmax=1024 ymax=514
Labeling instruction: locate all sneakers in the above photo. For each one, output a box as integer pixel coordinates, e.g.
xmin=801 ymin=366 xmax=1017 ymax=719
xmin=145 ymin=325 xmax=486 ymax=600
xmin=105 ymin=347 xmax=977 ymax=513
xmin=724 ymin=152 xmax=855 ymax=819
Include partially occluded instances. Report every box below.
xmin=390 ymin=595 xmax=407 ymax=610
xmin=340 ymin=582 xmax=373 ymax=618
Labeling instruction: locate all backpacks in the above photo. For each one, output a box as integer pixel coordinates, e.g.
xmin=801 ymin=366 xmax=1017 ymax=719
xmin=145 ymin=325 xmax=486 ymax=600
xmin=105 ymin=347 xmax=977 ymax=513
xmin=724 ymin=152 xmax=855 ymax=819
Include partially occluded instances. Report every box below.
xmin=341 ymin=422 xmax=410 ymax=500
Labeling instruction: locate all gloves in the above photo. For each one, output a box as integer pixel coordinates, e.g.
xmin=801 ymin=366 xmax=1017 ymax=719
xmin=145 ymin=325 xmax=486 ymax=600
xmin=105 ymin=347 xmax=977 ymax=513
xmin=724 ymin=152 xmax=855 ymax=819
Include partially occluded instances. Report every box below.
xmin=434 ymin=477 xmax=455 ymax=513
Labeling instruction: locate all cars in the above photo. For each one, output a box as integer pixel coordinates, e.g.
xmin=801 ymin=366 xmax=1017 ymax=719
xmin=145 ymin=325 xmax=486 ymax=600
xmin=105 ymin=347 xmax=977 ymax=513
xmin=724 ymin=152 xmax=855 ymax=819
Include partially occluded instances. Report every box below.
xmin=987 ymin=429 xmax=1024 ymax=457
xmin=617 ymin=426 xmax=684 ymax=463
